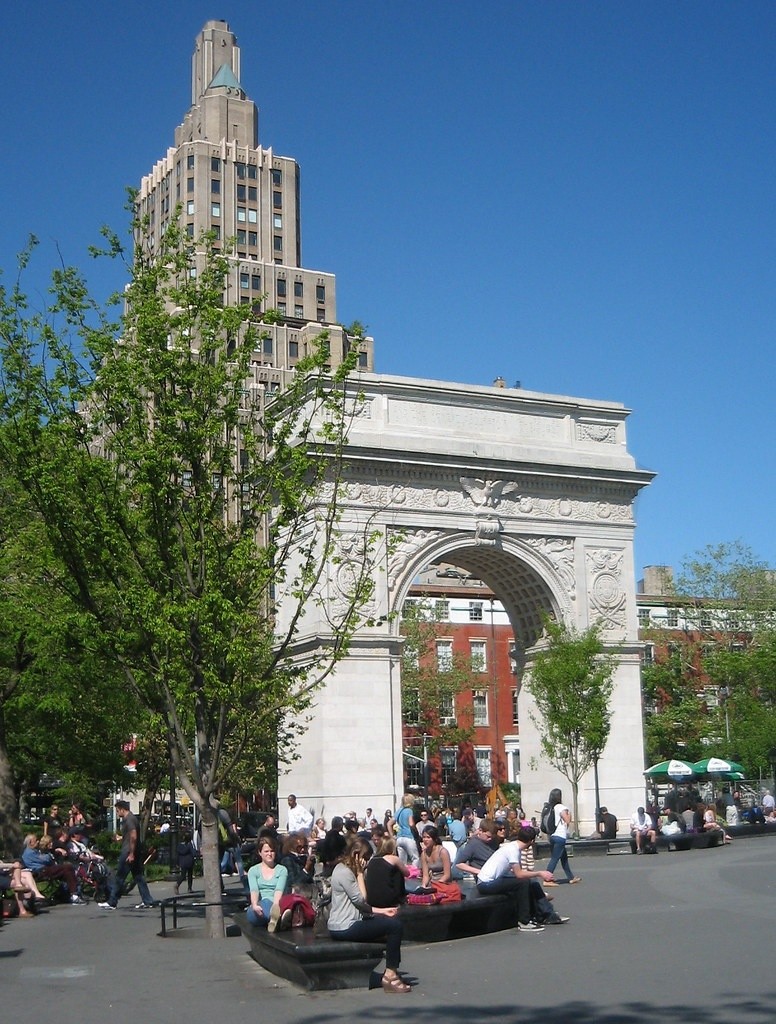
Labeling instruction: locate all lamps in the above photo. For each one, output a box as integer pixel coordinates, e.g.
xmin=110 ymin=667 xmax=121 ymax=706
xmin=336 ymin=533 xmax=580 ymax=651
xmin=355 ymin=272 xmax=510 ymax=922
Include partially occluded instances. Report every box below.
xmin=475 ymin=519 xmax=500 ymax=546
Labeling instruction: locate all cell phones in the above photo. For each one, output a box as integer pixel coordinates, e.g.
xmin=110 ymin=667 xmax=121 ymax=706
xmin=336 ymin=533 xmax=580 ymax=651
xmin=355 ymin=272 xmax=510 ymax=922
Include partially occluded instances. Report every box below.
xmin=353 ymin=850 xmax=360 ymax=860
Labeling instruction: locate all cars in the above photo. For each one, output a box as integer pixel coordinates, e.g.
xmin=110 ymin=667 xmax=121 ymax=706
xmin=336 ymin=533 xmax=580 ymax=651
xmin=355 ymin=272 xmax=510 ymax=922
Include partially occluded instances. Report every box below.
xmin=21 ymin=794 xmax=279 ymax=844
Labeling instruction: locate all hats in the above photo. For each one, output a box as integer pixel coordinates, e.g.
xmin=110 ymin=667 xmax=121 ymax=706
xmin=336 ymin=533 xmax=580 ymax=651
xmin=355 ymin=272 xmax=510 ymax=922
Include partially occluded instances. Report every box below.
xmin=69 ymin=827 xmax=81 ymax=835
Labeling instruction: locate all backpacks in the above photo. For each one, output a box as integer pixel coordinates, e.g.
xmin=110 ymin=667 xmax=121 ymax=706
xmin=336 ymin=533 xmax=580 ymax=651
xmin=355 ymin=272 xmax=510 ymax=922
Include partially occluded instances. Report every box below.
xmin=540 ymin=806 xmax=562 ymax=835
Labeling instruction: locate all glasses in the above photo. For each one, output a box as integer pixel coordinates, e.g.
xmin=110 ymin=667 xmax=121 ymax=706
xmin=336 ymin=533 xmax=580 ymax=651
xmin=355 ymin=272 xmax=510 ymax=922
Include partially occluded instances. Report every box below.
xmin=53 ymin=809 xmax=58 ymax=810
xmin=420 ymin=814 xmax=427 ymax=817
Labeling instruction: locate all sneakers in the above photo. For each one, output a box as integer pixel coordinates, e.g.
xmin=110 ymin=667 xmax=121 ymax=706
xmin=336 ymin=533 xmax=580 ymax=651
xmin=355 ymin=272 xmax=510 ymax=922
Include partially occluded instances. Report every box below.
xmin=136 ymin=902 xmax=153 ymax=909
xmin=98 ymin=902 xmax=116 ymax=910
xmin=518 ymin=921 xmax=546 ymax=931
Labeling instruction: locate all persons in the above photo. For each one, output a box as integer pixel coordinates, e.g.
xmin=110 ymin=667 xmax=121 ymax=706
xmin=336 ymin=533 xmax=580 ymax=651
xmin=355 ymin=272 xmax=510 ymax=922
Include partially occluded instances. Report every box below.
xmin=327 ymin=837 xmax=411 ymax=993
xmin=658 ymin=787 xmax=776 ymax=845
xmin=543 ymin=788 xmax=581 ymax=886
xmin=0 ymin=821 xmax=137 ymax=918
xmin=248 ymin=794 xmax=570 ymax=933
xmin=97 ymin=801 xmax=154 ymax=908
xmin=43 ymin=804 xmax=62 ymax=836
xmin=630 ymin=806 xmax=658 ymax=855
xmin=159 ymin=809 xmax=247 ymax=895
xmin=68 ymin=803 xmax=87 ymax=827
xmin=598 ymin=806 xmax=618 ymax=839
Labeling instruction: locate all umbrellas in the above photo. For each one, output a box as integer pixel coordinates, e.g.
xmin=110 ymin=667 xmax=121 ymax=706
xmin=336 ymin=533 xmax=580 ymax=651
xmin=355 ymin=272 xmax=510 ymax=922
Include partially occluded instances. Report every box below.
xmin=644 ymin=759 xmax=746 ymax=792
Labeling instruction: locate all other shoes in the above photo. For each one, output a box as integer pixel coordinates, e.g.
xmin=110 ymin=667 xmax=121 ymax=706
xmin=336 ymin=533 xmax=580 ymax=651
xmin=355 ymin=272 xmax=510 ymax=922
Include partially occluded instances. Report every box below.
xmin=267 ymin=904 xmax=280 ymax=933
xmin=637 ymin=849 xmax=642 ymax=855
xmin=174 ymin=885 xmax=180 ymax=895
xmin=547 ymin=915 xmax=571 ymax=924
xmin=543 ymin=881 xmax=559 ymax=887
xmin=569 ymin=877 xmax=581 ymax=884
xmin=10 ymin=886 xmax=96 ymax=918
xmin=726 ymin=835 xmax=733 ymax=840
xmin=545 ymin=892 xmax=553 ymax=901
xmin=650 ymin=849 xmax=659 ymax=854
xmin=281 ymin=909 xmax=293 ymax=931
xmin=188 ymin=889 xmax=195 ymax=893
xmin=725 ymin=841 xmax=731 ymax=844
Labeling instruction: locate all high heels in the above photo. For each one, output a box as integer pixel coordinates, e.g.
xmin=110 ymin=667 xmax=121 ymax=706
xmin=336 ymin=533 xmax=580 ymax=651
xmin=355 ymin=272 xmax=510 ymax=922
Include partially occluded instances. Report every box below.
xmin=382 ymin=976 xmax=411 ymax=993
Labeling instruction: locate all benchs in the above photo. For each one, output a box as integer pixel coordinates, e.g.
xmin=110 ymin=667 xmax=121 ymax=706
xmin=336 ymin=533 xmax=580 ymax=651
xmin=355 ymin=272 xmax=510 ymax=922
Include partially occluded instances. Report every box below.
xmin=533 ymin=819 xmax=776 ymax=859
xmin=234 ymin=912 xmax=388 ymax=993
xmin=392 ymin=881 xmax=518 ymax=946
xmin=0 ymin=869 xmax=90 ymax=906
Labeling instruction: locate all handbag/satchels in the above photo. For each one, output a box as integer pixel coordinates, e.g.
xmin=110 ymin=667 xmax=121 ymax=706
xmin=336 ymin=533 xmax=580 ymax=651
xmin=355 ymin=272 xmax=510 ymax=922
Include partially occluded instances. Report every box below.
xmin=431 ymin=880 xmax=462 ymax=903
xmin=407 ymin=893 xmax=448 ymax=906
xmin=313 ymin=895 xmax=333 ymax=938
